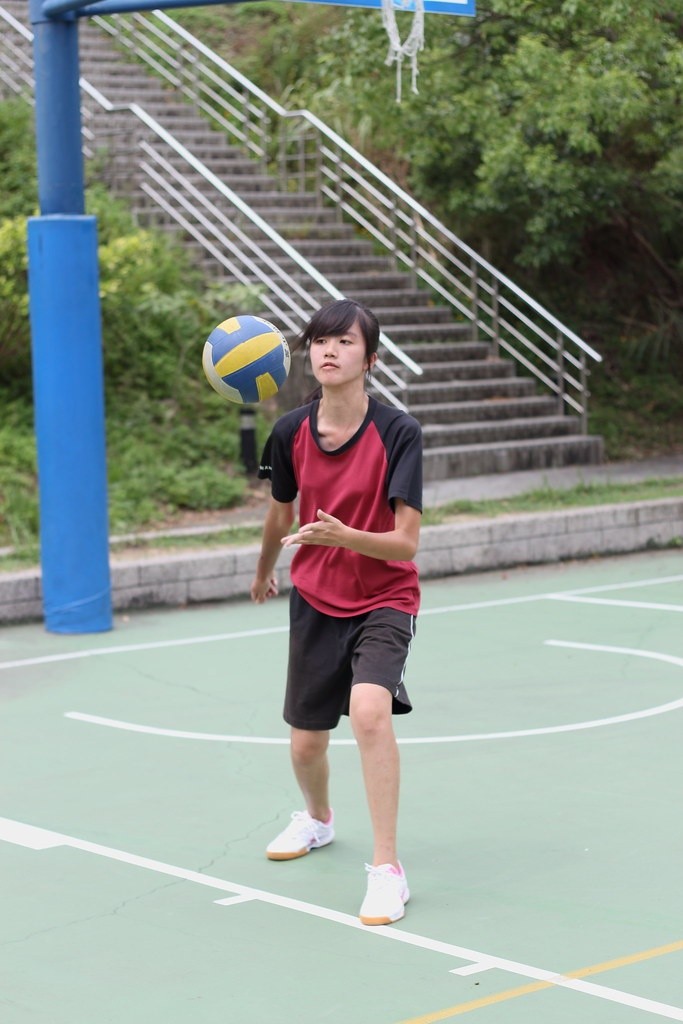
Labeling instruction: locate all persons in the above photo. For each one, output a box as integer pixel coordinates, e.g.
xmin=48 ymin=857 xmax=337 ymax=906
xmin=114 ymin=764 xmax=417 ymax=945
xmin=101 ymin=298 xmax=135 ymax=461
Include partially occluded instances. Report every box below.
xmin=249 ymin=299 xmax=424 ymax=926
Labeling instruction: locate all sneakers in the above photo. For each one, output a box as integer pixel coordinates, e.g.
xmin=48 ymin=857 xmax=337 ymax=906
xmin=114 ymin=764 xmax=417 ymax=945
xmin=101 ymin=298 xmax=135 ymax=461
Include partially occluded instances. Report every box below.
xmin=360 ymin=859 xmax=411 ymax=926
xmin=266 ymin=808 xmax=336 ymax=860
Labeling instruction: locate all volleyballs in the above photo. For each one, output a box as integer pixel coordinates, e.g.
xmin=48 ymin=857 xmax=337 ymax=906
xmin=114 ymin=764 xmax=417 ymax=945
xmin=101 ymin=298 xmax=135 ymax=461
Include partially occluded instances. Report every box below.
xmin=201 ymin=314 xmax=292 ymax=405
